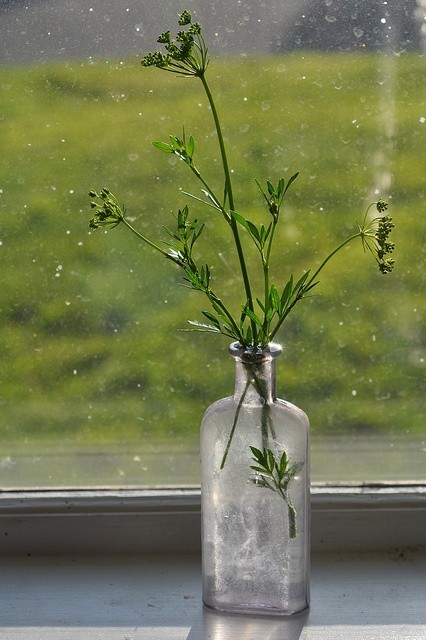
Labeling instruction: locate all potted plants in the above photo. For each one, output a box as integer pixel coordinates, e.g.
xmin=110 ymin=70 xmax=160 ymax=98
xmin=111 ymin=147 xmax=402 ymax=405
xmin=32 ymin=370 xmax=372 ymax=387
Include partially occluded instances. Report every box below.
xmin=90 ymin=11 xmax=395 ymax=617
